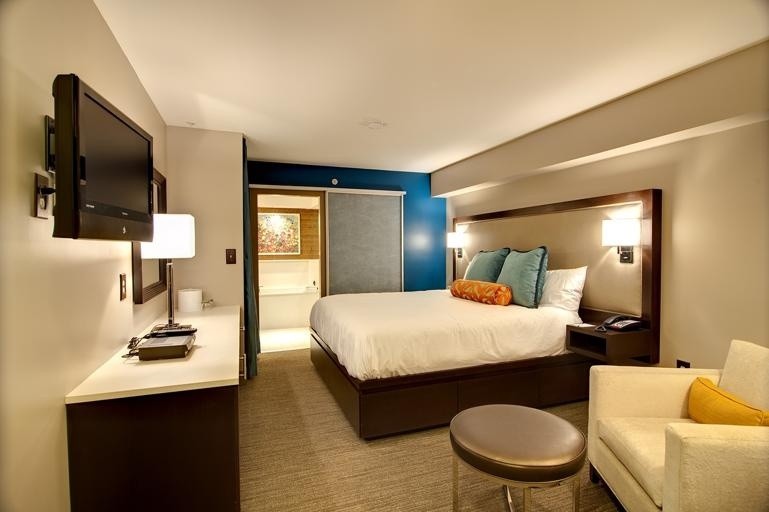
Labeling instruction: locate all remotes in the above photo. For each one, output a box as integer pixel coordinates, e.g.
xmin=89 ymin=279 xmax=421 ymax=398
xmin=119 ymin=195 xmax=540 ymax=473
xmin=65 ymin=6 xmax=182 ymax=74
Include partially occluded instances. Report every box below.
xmin=151 ymin=327 xmax=197 ymax=337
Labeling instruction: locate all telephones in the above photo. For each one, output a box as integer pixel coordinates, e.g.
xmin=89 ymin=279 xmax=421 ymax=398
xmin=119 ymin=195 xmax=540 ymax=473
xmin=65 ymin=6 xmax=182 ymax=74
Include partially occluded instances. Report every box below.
xmin=603 ymin=315 xmax=641 ymax=331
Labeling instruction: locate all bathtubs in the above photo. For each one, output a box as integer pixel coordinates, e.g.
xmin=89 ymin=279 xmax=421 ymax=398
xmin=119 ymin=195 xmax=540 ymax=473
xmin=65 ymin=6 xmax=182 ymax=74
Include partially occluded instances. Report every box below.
xmin=259 ymin=284 xmax=319 ymax=296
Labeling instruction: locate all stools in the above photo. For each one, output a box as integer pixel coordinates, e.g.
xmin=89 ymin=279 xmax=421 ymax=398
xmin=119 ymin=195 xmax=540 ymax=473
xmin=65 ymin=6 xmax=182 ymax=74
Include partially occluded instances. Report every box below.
xmin=449 ymin=402 xmax=587 ymax=512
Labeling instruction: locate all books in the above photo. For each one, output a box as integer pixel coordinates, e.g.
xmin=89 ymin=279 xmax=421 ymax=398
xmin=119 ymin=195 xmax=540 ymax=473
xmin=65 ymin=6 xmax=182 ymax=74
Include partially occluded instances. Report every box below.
xmin=138 ymin=335 xmax=195 ymax=358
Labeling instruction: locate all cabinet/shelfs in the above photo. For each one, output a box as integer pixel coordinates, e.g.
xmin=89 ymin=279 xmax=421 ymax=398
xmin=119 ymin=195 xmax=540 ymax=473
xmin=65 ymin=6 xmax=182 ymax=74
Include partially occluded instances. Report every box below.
xmin=63 ymin=303 xmax=247 ymax=512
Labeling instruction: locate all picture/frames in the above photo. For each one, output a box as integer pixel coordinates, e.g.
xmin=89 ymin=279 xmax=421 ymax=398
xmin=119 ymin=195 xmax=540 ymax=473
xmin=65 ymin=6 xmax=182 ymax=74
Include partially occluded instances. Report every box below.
xmin=132 ymin=168 xmax=169 ymax=307
xmin=257 ymin=212 xmax=302 ymax=257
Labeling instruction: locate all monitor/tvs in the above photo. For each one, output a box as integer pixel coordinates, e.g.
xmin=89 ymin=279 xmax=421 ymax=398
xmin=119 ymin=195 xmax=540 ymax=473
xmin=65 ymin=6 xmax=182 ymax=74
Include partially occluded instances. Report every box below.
xmin=51 ymin=72 xmax=154 ymax=244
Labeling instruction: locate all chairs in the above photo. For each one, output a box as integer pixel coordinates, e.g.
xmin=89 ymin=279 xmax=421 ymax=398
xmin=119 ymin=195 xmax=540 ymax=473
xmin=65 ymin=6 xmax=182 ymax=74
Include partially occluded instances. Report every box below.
xmin=587 ymin=338 xmax=769 ymax=512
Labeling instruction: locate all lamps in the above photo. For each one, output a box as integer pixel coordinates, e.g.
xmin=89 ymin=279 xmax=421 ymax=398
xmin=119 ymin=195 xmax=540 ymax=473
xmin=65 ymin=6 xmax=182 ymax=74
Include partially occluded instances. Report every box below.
xmin=445 ymin=230 xmax=467 ymax=259
xmin=140 ymin=212 xmax=198 ymax=339
xmin=601 ymin=216 xmax=640 ymax=265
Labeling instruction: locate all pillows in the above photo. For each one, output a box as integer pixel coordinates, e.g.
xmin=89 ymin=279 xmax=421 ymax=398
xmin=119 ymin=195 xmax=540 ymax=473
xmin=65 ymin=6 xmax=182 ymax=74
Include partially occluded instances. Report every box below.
xmin=538 ymin=266 xmax=589 ymax=313
xmin=496 ymin=244 xmax=551 ymax=309
xmin=464 ymin=247 xmax=511 ymax=283
xmin=451 ymin=278 xmax=513 ymax=306
xmin=689 ymin=376 xmax=769 ymax=428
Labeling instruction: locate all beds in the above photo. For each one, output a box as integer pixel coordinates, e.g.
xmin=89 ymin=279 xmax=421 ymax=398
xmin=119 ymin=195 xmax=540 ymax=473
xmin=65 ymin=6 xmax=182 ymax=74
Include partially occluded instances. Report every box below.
xmin=307 ymin=187 xmax=663 ymax=443
